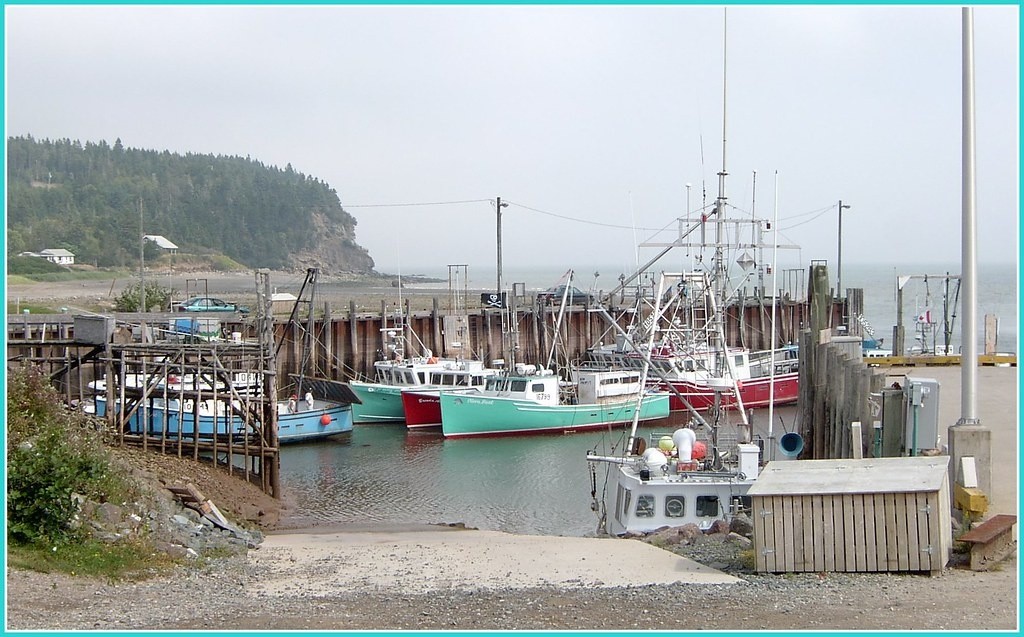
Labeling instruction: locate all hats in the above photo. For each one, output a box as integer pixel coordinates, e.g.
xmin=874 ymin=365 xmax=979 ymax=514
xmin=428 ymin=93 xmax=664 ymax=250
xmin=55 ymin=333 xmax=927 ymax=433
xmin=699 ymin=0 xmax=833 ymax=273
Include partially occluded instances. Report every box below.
xmin=290 ymin=395 xmax=297 ymax=399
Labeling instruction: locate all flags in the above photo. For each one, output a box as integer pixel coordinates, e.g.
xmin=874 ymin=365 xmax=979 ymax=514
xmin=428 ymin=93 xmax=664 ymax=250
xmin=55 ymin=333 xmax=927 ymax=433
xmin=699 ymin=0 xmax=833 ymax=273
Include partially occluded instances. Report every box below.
xmin=917 ymin=310 xmax=931 ymax=323
xmin=481 ymin=293 xmax=507 ymax=307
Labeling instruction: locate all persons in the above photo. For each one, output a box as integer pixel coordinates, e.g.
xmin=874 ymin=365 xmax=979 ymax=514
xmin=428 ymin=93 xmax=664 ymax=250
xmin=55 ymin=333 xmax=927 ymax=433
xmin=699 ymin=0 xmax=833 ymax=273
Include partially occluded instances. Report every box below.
xmin=305 ymin=388 xmax=314 ymax=410
xmin=287 ymin=399 xmax=297 ymax=412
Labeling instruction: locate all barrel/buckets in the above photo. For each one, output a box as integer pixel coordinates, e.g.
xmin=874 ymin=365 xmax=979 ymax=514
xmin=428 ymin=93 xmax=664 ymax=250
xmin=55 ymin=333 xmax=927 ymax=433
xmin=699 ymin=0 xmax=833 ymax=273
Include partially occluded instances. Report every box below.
xmin=232 ymin=332 xmax=241 ymax=343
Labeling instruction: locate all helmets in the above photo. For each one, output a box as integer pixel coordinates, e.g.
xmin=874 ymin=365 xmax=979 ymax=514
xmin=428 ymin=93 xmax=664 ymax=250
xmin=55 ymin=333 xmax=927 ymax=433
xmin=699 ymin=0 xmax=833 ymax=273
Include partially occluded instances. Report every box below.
xmin=309 ymin=388 xmax=313 ymax=391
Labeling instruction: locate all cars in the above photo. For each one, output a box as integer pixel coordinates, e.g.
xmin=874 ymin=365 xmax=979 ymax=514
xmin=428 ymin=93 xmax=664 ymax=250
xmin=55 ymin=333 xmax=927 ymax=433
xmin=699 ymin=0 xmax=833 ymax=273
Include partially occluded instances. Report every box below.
xmin=534 ymin=285 xmax=596 ymax=308
xmin=171 ymin=295 xmax=249 ymax=314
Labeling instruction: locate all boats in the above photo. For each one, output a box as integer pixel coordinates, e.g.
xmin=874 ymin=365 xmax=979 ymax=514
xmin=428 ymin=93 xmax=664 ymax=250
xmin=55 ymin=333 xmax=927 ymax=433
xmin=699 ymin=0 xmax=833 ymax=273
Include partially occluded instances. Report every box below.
xmin=585 ymin=165 xmax=781 ymax=541
xmin=66 ymin=4 xmax=963 ymax=450
